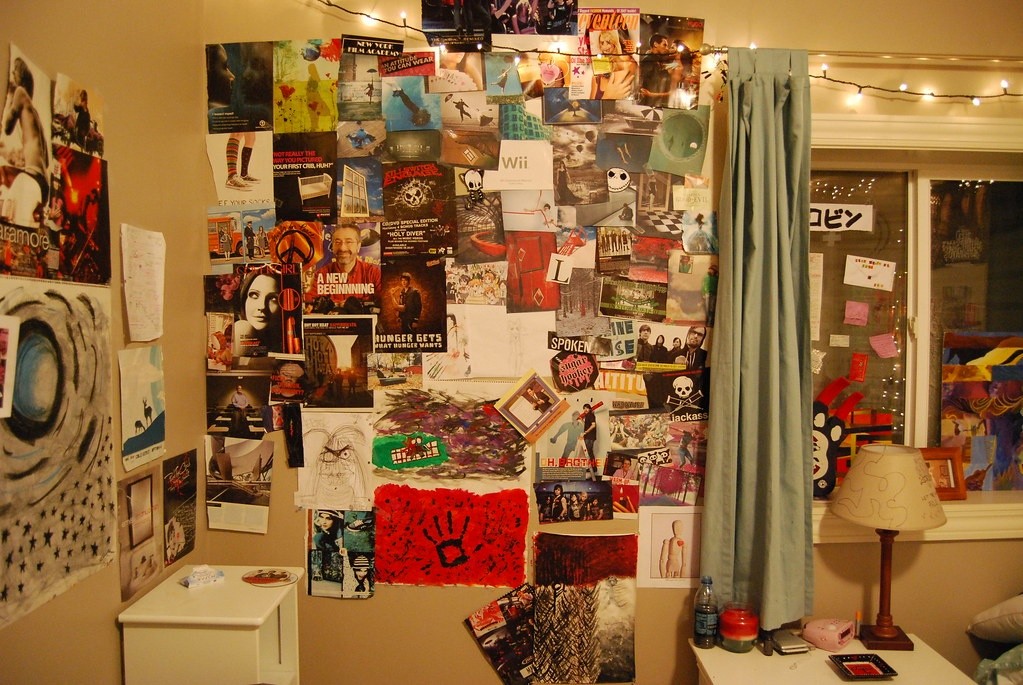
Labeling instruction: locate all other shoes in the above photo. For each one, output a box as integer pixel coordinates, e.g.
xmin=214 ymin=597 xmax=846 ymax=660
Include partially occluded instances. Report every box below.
xmin=586 ymin=466 xmax=597 ymax=472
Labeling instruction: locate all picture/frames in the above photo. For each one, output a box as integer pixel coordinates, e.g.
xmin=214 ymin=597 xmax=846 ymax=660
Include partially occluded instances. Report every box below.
xmin=918 ymin=447 xmax=967 ymax=500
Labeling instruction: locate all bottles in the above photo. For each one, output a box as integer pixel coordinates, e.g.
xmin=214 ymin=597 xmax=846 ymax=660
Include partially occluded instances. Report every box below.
xmin=763 ymin=635 xmax=773 ymax=656
xmin=718 ymin=603 xmax=760 ymax=653
xmin=694 ymin=576 xmax=718 ymax=649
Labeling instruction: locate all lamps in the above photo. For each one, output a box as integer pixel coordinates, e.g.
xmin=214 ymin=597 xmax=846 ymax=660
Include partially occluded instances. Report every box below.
xmin=830 ymin=444 xmax=948 ymax=650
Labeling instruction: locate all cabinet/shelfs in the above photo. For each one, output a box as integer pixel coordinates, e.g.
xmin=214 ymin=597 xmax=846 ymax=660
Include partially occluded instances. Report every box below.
xmin=117 ymin=563 xmax=305 ymax=685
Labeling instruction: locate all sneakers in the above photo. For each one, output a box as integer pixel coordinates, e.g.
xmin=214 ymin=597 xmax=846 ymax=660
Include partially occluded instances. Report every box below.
xmin=225 ymin=174 xmax=254 ymax=190
xmin=240 ymin=174 xmax=261 ymax=184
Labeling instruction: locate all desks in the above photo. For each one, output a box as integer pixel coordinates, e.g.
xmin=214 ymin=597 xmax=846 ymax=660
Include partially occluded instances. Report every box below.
xmin=688 ymin=633 xmax=980 ymax=685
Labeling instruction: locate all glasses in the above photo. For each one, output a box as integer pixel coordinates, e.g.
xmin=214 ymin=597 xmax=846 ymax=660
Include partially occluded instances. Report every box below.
xmin=691 ymin=330 xmax=706 ymax=338
xmin=331 ymin=239 xmax=358 ymax=246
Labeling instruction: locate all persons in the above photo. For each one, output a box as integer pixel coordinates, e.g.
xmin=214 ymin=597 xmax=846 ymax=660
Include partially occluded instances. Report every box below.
xmin=207 ymin=0 xmax=720 ymax=598
xmin=0 ymin=56 xmax=92 ymax=276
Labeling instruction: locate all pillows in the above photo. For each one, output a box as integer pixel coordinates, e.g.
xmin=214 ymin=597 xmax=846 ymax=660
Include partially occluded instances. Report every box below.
xmin=964 ymin=594 xmax=1023 ymax=643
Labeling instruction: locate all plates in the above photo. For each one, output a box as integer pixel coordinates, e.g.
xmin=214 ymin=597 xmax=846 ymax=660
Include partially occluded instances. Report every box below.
xmin=828 ymin=653 xmax=899 ymax=680
